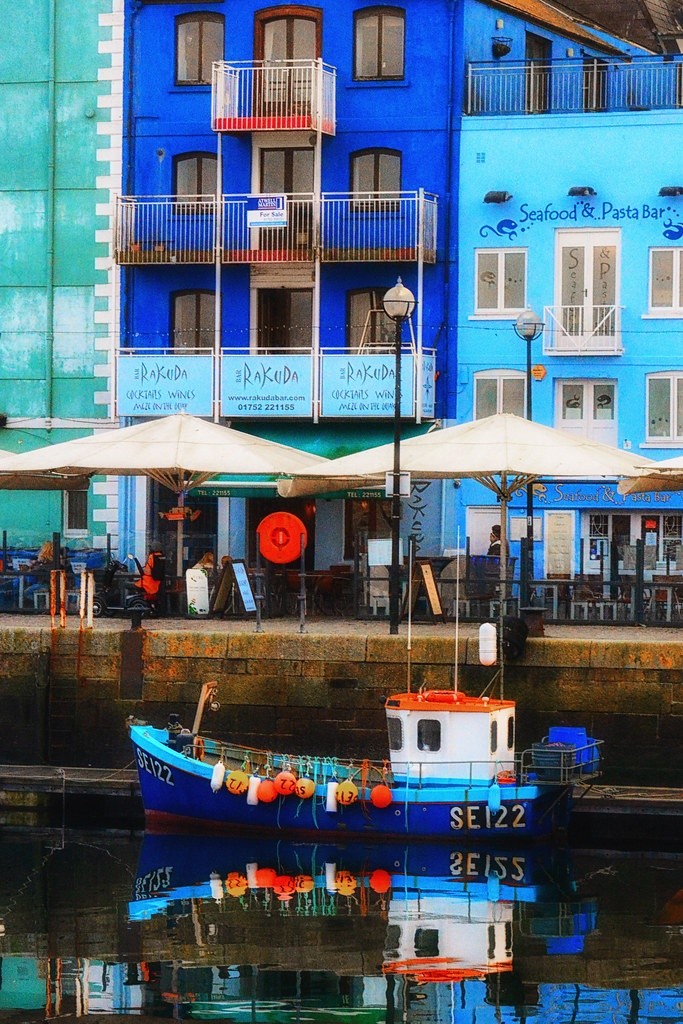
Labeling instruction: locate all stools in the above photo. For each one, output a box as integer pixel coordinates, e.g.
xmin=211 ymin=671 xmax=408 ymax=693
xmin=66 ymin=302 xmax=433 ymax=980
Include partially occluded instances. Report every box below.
xmin=66 ymin=589 xmax=81 ymax=612
xmin=452 ymin=595 xmax=518 ymax=618
xmin=571 ymin=599 xmax=683 ymax=621
xmin=34 ymin=589 xmax=49 ymax=609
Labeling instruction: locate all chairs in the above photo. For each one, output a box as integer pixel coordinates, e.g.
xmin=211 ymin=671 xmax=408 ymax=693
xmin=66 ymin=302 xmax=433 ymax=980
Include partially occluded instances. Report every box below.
xmin=251 ymin=564 xmax=361 ymax=618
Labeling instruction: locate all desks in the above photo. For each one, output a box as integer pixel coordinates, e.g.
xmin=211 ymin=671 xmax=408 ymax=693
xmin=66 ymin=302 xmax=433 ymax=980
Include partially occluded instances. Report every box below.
xmin=631 ymin=583 xmax=683 ymax=622
xmin=529 ymin=579 xmax=574 ymax=619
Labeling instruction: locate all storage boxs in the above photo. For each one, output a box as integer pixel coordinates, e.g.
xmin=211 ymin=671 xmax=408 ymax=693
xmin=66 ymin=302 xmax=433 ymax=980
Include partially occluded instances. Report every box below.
xmin=549 ymin=727 xmax=599 ymax=773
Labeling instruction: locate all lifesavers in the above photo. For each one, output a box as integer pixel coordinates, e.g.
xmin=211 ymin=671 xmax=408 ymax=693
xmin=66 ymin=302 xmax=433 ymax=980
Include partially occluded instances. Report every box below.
xmin=423 ymin=689 xmax=465 ymax=704
xmin=194 ymin=737 xmax=205 ymax=761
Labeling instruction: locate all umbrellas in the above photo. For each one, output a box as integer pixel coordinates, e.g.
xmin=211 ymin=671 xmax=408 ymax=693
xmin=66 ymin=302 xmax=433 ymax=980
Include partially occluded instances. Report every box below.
xmin=272 ymin=413 xmax=682 ymax=617
xmin=0 ymin=408 xmax=332 ymax=577
xmin=0 ymin=449 xmax=91 ymax=490
xmin=617 ymin=453 xmax=683 ymax=497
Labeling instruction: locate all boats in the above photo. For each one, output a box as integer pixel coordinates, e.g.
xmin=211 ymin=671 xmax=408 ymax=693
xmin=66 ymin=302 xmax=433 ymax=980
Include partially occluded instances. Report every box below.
xmin=121 ymin=711 xmax=606 ymax=847
xmin=133 ymin=832 xmax=553 ymax=987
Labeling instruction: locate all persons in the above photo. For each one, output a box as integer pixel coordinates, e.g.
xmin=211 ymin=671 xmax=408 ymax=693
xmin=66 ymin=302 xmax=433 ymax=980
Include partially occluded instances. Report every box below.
xmin=0 ymin=540 xmax=74 ymax=604
xmin=487 ymin=521 xmax=510 ymax=563
xmin=133 ymin=541 xmax=165 ymax=603
xmin=189 ymin=551 xmax=233 ymax=576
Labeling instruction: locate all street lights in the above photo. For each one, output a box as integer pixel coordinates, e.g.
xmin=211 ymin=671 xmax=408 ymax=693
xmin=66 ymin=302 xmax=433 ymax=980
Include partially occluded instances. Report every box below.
xmin=381 ymin=277 xmax=417 ymax=637
xmin=510 ymin=303 xmax=547 ymax=576
